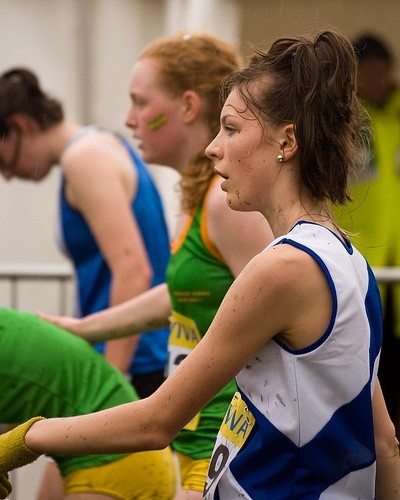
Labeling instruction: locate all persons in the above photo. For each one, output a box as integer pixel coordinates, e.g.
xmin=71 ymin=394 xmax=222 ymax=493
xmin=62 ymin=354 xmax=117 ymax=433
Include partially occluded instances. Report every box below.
xmin=2 ymin=68 xmax=171 ymax=500
xmin=326 ymin=32 xmax=400 ymax=455
xmin=0 ymin=307 xmax=177 ymax=500
xmin=35 ymin=30 xmax=275 ymax=500
xmin=1 ymin=30 xmax=399 ymax=500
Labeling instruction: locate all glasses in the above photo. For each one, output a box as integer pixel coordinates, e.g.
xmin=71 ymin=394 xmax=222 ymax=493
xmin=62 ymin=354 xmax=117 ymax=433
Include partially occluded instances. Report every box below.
xmin=2 ymin=132 xmax=22 ymax=179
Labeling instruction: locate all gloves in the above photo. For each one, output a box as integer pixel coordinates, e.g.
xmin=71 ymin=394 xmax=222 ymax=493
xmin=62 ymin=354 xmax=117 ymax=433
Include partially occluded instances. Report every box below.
xmin=0 ymin=416 xmax=46 ymax=500
